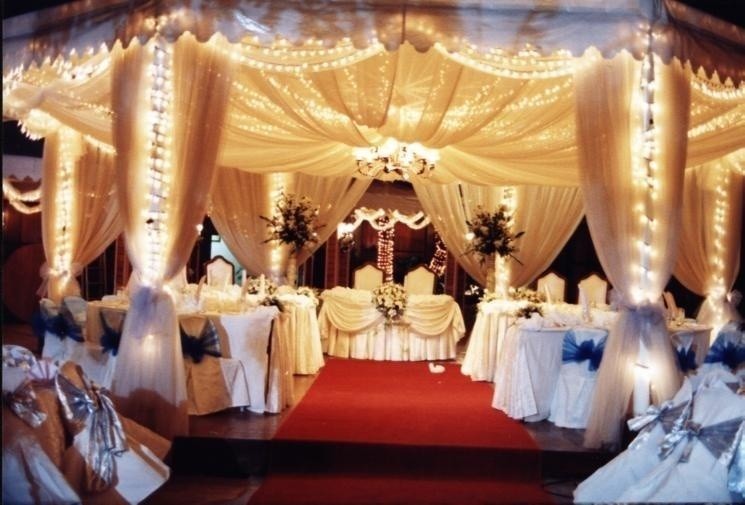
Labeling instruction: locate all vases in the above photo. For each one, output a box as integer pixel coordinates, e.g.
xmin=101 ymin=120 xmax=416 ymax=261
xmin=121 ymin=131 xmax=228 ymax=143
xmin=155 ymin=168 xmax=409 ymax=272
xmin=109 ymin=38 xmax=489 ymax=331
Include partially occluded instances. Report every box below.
xmin=487 ymin=252 xmax=495 ymax=294
xmin=286 ymin=242 xmax=299 ymax=290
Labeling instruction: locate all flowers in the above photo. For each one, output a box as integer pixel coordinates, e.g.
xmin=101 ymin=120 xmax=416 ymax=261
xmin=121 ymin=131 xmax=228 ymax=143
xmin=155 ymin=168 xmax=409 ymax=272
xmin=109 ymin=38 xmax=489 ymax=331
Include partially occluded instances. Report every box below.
xmin=458 ymin=203 xmax=526 ymax=267
xmin=260 ymin=191 xmax=327 ymax=258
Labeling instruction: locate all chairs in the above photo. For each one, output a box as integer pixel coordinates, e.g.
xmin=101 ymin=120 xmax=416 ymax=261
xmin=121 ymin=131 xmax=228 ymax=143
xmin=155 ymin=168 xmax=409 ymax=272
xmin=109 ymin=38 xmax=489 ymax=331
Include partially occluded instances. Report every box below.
xmin=575 ymin=273 xmax=610 ymax=307
xmin=403 ymin=263 xmax=438 ymax=297
xmin=536 ymin=269 xmax=568 ymax=302
xmin=203 ymin=255 xmax=235 ymax=289
xmin=350 ymin=262 xmax=386 ymax=288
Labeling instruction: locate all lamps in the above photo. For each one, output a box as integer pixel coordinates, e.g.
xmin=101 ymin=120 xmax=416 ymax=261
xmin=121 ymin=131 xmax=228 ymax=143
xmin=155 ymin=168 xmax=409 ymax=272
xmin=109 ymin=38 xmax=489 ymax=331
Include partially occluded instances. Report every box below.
xmin=353 ymin=141 xmax=441 ymax=178
